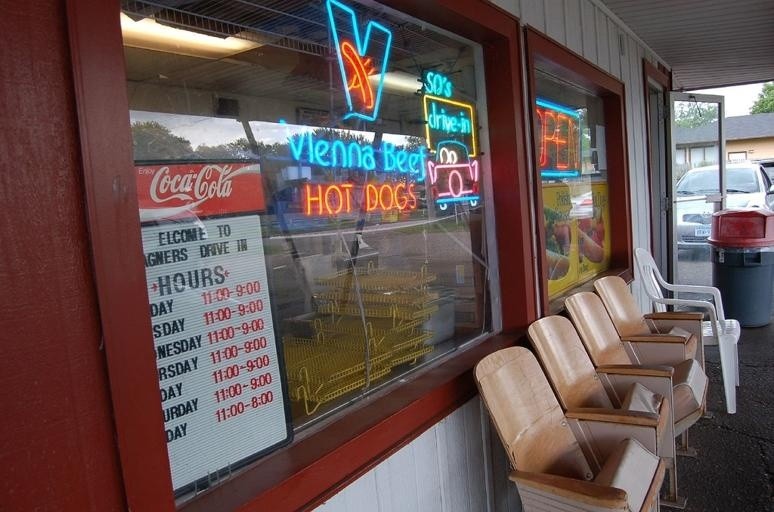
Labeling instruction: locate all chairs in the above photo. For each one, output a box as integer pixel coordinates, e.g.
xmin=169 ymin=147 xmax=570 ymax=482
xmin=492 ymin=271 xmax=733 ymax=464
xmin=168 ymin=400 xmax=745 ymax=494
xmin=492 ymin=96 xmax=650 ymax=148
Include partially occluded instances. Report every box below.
xmin=473 ymin=247 xmax=741 ymax=512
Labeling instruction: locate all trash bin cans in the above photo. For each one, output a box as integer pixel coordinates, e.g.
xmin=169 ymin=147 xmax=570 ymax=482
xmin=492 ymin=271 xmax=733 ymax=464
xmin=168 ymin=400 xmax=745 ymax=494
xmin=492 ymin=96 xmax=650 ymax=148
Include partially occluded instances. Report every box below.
xmin=707 ymin=208 xmax=774 ymax=327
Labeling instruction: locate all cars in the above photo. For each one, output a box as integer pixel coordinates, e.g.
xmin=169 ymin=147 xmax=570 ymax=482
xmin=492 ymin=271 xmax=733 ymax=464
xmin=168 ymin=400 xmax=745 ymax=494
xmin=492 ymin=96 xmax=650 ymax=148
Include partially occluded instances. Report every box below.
xmin=672 ymin=162 xmax=774 ymax=261
